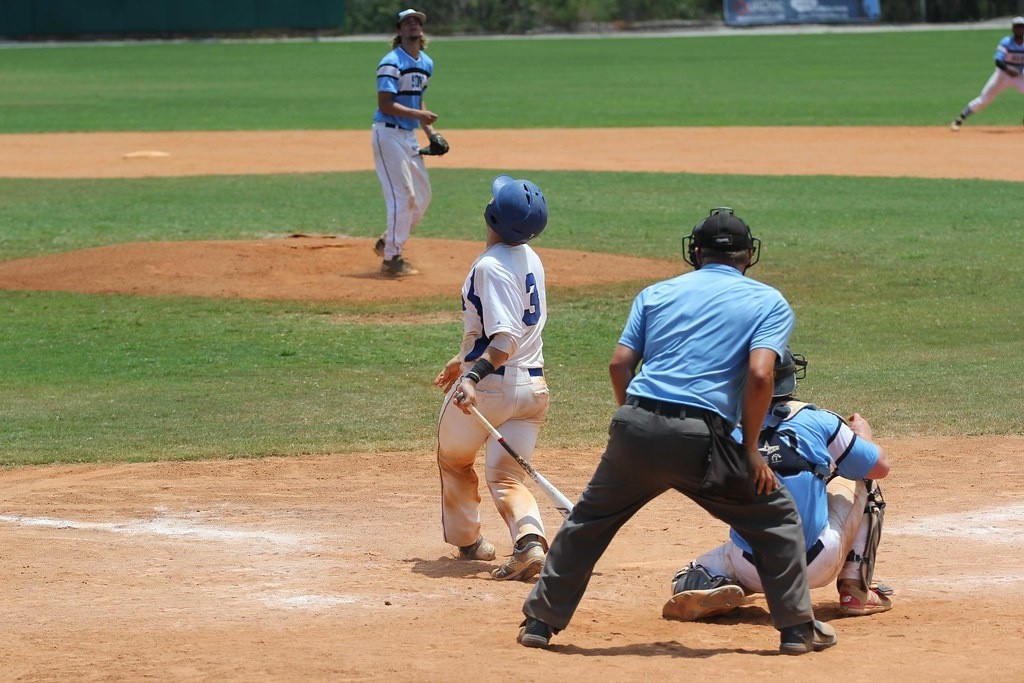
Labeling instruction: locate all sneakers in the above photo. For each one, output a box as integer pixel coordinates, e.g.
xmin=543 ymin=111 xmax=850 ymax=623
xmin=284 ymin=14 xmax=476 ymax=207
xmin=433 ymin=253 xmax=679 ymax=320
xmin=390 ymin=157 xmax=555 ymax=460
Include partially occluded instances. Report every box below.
xmin=518 ymin=618 xmax=552 ymax=648
xmin=458 ymin=534 xmax=497 ymax=561
xmin=662 ymin=584 xmax=745 ymax=621
xmin=838 ymin=583 xmax=893 ymax=615
xmin=780 ymin=625 xmax=838 ymax=653
xmin=490 ymin=541 xmax=545 ymax=581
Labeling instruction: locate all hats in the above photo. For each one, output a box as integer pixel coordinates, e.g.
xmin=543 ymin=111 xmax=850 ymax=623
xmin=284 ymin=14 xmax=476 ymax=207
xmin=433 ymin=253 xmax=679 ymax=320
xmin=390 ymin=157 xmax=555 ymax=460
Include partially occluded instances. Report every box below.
xmin=395 ymin=9 xmax=427 ymax=32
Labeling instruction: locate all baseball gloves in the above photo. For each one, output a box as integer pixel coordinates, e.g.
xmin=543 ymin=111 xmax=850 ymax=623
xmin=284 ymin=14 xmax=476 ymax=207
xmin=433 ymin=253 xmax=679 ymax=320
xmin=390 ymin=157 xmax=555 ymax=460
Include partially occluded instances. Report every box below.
xmin=417 ymin=132 xmax=450 ymax=156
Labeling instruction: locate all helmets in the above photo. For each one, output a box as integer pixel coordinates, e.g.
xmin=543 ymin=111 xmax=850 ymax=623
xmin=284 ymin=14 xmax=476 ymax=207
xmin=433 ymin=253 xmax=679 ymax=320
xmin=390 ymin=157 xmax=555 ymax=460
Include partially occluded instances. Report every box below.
xmin=694 ymin=212 xmax=753 ymax=253
xmin=1012 ymin=17 xmax=1024 ymax=33
xmin=485 ymin=174 xmax=548 ymax=244
xmin=771 ymin=344 xmax=798 ymax=397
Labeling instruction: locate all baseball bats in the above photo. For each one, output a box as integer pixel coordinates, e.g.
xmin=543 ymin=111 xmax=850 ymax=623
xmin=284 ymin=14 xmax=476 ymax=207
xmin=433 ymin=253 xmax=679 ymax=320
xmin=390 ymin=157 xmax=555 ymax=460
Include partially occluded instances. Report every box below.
xmin=456 ymin=392 xmax=575 ymax=519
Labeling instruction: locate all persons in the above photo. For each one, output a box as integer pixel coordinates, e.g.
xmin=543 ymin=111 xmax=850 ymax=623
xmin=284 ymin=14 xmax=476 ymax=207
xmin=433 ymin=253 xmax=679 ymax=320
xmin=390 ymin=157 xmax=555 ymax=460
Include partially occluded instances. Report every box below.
xmin=951 ymin=15 xmax=1024 ymax=129
xmin=432 ymin=175 xmax=549 ymax=581
xmin=662 ymin=347 xmax=893 ymax=623
xmin=370 ymin=8 xmax=449 ymax=279
xmin=517 ymin=206 xmax=838 ymax=654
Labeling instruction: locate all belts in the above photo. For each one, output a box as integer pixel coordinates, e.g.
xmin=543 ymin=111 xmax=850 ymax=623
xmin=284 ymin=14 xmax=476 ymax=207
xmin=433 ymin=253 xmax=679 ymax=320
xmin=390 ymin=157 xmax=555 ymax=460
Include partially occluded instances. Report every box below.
xmin=743 ymin=537 xmax=824 ymax=568
xmin=491 ymin=365 xmax=545 ymax=377
xmin=625 ymin=396 xmax=735 ymax=435
xmin=385 ymin=122 xmax=405 ymax=130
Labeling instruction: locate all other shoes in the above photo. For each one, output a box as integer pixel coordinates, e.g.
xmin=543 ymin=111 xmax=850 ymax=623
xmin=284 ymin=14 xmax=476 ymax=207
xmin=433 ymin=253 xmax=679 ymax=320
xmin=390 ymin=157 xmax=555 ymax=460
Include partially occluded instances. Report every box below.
xmin=951 ymin=118 xmax=964 ymax=130
xmin=381 ymin=255 xmax=420 ymax=277
xmin=374 ymin=239 xmax=387 ymax=258
